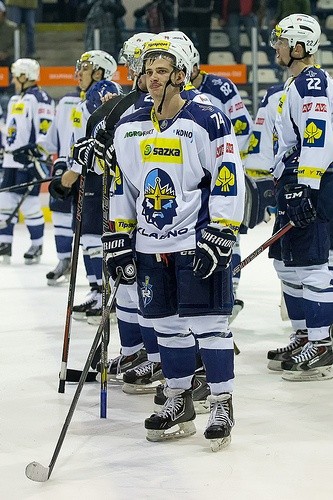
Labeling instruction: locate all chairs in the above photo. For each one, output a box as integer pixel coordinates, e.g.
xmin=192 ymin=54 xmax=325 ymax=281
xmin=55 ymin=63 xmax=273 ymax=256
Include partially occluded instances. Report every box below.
xmin=206 ymin=0 xmax=333 ymax=119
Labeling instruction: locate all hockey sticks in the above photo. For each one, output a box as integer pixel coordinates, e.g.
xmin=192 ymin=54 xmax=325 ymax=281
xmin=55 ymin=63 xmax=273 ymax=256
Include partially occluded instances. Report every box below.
xmin=57 ymin=94 xmax=124 ymax=394
xmin=0 ymin=178 xmax=37 ymax=229
xmin=230 ymin=219 xmax=296 ymax=277
xmin=99 ymin=90 xmax=139 ymax=418
xmin=25 ymin=270 xmax=123 ymax=483
xmin=0 ymin=175 xmax=60 ymax=193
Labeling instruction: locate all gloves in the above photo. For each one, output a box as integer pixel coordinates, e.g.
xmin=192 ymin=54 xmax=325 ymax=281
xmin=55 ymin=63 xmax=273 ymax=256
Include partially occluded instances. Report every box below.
xmin=48 ymin=156 xmax=72 ymax=198
xmin=12 ymin=144 xmax=43 ymax=164
xmin=192 ymin=224 xmax=237 ymax=280
xmin=100 ymin=232 xmax=138 ymax=286
xmin=283 ymin=184 xmax=319 ymax=230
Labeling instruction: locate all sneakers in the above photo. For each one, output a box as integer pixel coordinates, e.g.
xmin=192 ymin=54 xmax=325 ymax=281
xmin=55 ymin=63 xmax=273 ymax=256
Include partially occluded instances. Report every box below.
xmin=122 ymin=361 xmax=165 ymax=395
xmin=45 ymin=258 xmax=72 ymax=286
xmin=267 ymin=329 xmax=319 ymax=371
xmin=96 ymin=347 xmax=148 ymax=385
xmin=202 ymin=393 xmax=235 ymax=453
xmin=280 ymin=340 xmax=333 ymax=381
xmin=71 ymin=287 xmax=102 ymax=321
xmin=24 ymin=245 xmax=43 ymax=266
xmin=0 ymin=243 xmax=13 ymax=265
xmin=154 ymin=372 xmax=211 ymax=415
xmin=85 ymin=292 xmax=118 ymax=325
xmin=144 ymin=386 xmax=197 ymax=442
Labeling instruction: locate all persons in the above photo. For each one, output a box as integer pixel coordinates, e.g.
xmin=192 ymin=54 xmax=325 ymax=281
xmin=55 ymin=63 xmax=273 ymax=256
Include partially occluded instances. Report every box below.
xmin=41 ymin=49 xmax=126 ymax=323
xmin=5 ymin=0 xmax=39 ymax=58
xmin=253 ymin=14 xmax=333 ymax=381
xmin=0 ymin=58 xmax=54 ymax=266
xmin=0 ymin=0 xmax=26 ymax=67
xmin=71 ymin=30 xmax=253 ymax=453
xmin=84 ymin=0 xmax=333 ymax=64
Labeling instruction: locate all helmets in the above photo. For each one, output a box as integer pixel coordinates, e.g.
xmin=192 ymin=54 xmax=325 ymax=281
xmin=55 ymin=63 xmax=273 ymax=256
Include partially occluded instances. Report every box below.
xmin=140 ymin=38 xmax=193 ymax=89
xmin=151 ymin=29 xmax=201 ymax=67
xmin=10 ymin=58 xmax=41 ymax=82
xmin=269 ymin=13 xmax=322 ymax=55
xmin=73 ymin=49 xmax=118 ymax=81
xmin=117 ymin=32 xmax=158 ymax=80
